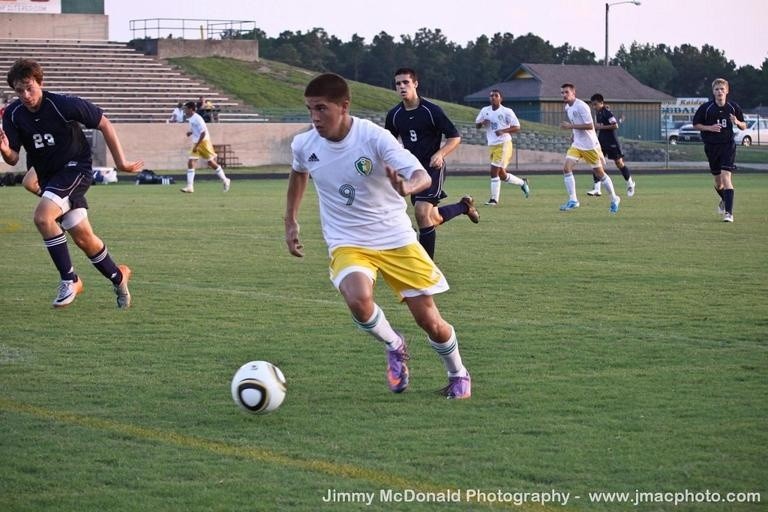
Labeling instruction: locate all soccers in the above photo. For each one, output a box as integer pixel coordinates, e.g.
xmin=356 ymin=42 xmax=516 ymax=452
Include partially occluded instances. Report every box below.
xmin=231 ymin=360 xmax=286 ymax=415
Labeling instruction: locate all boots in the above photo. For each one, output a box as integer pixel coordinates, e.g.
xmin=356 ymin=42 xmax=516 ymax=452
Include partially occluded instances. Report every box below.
xmin=625 ymin=176 xmax=635 ymax=197
xmin=587 ymin=182 xmax=602 ymax=196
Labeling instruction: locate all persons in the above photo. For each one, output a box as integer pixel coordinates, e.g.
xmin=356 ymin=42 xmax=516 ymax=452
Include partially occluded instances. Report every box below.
xmin=284 ymin=73 xmax=472 ymax=401
xmin=558 ymin=83 xmax=621 ymax=214
xmin=197 ymin=95 xmax=210 ymax=122
xmin=0 ymin=58 xmax=145 ymax=309
xmin=383 ymin=69 xmax=480 ymax=261
xmin=475 ymin=88 xmax=531 ymax=207
xmin=586 ymin=93 xmax=635 ymax=198
xmin=0 ymin=93 xmax=20 ymax=117
xmin=170 ymin=103 xmax=184 ymax=123
xmin=179 ymin=101 xmax=230 ymax=194
xmin=693 ymin=77 xmax=746 ymax=223
xmin=21 ymin=152 xmax=65 ymax=233
xmin=205 ymin=100 xmax=220 ymax=123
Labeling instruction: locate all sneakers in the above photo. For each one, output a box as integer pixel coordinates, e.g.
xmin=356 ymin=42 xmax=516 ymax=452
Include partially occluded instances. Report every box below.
xmin=387 ymin=331 xmax=411 ymax=393
xmin=460 ymin=195 xmax=480 ymax=223
xmin=521 ymin=178 xmax=529 ymax=198
xmin=611 ymin=196 xmax=620 ymax=212
xmin=718 ymin=200 xmax=725 ymax=215
xmin=224 ymin=178 xmax=230 ymax=193
xmin=485 ymin=199 xmax=497 ymax=205
xmin=560 ymin=200 xmax=579 ymax=211
xmin=434 ymin=369 xmax=471 ymax=399
xmin=181 ymin=187 xmax=194 ymax=193
xmin=53 ymin=276 xmax=82 ymax=308
xmin=724 ymin=212 xmax=733 ymax=222
xmin=114 ymin=265 xmax=131 ymax=308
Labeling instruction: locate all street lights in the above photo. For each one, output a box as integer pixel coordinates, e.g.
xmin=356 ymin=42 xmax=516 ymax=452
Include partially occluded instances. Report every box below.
xmin=605 ymin=1 xmax=642 ymax=66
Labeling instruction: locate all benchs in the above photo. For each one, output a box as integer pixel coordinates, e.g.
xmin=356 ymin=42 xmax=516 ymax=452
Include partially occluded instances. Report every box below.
xmin=0 ymin=38 xmax=269 ymax=123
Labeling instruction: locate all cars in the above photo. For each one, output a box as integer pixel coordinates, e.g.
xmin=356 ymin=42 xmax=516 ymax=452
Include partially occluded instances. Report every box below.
xmin=733 ymin=119 xmax=767 ymax=146
xmin=660 ymin=123 xmax=703 ymax=145
